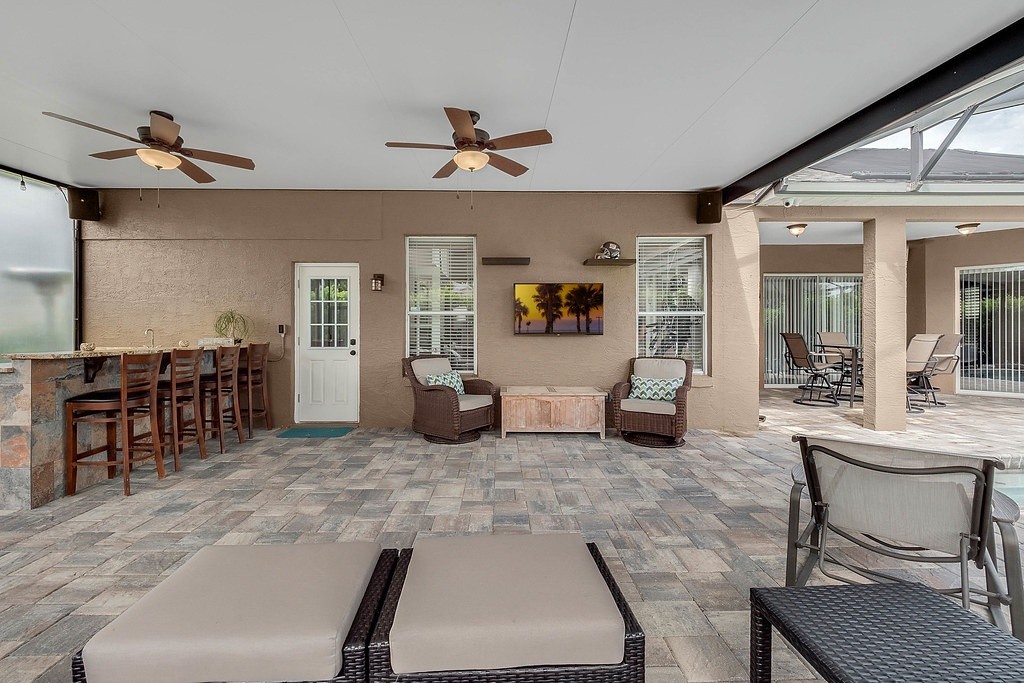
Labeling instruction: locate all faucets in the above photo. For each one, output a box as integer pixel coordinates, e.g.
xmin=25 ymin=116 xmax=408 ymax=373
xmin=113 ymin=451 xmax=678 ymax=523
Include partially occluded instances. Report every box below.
xmin=142 ymin=328 xmax=154 ymax=348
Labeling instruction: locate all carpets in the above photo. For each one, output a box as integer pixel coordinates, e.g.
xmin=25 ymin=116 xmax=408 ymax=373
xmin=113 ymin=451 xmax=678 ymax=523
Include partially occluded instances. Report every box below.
xmin=275 ymin=426 xmax=354 ymax=438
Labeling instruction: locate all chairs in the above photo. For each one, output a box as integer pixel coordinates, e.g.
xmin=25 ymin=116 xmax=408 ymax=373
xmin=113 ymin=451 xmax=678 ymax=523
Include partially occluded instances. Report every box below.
xmin=779 ymin=331 xmax=966 ymax=413
xmin=611 ymin=356 xmax=694 ymax=449
xmin=401 ymin=354 xmax=497 ymax=445
xmin=785 ymin=434 xmax=1024 ymax=641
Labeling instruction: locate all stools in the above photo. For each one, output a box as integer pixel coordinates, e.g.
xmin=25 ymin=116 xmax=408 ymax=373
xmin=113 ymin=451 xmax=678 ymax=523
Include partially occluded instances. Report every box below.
xmin=63 ymin=343 xmax=271 ymax=496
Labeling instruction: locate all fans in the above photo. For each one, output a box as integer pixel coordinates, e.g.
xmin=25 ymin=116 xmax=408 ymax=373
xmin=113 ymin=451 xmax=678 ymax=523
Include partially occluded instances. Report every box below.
xmin=385 ymin=107 xmax=553 ymax=179
xmin=41 ymin=110 xmax=255 ymax=183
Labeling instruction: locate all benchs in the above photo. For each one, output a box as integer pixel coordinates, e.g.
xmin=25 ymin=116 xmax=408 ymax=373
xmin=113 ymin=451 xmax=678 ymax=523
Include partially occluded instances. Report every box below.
xmin=367 ymin=532 xmax=645 ymax=682
xmin=749 ymin=583 xmax=1024 ymax=683
xmin=68 ymin=539 xmax=399 ymax=683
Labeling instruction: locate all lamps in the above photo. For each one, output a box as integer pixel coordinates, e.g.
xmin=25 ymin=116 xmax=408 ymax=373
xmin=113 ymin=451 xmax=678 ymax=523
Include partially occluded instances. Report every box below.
xmin=453 ymin=146 xmax=490 ymax=210
xmin=787 ymin=224 xmax=808 ymax=237
xmin=371 ymin=273 xmax=385 ymax=291
xmin=955 ymin=223 xmax=980 ymax=236
xmin=136 ymin=145 xmax=182 ymax=208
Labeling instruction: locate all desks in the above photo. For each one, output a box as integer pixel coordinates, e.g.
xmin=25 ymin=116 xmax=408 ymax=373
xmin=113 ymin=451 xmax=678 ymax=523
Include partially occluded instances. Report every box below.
xmin=815 ymin=343 xmax=863 ymax=409
xmin=499 ymin=385 xmax=608 ymax=440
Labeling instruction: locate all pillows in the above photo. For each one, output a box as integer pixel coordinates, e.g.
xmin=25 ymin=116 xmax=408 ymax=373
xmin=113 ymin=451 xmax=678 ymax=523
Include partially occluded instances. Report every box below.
xmin=425 ymin=370 xmax=465 ymax=396
xmin=627 ymin=374 xmax=684 ymax=401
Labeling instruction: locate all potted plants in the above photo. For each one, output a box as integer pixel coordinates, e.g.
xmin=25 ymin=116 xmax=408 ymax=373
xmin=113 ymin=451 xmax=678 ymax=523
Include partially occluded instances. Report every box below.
xmin=213 ymin=308 xmax=255 ymax=345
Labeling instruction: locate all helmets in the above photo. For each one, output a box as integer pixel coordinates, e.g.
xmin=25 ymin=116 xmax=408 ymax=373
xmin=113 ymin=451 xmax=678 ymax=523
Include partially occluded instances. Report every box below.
xmin=594 ymin=241 xmax=621 ymax=260
